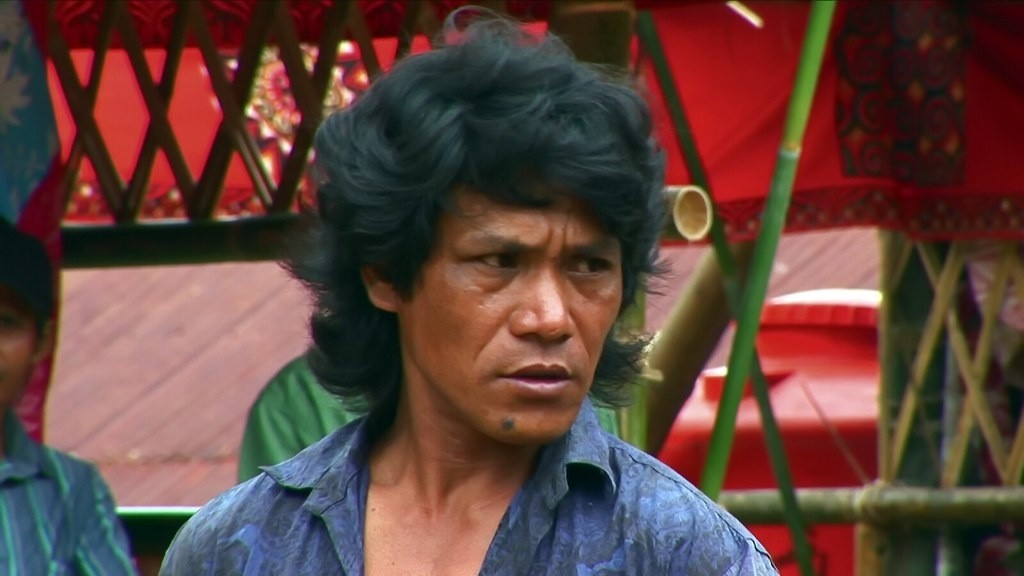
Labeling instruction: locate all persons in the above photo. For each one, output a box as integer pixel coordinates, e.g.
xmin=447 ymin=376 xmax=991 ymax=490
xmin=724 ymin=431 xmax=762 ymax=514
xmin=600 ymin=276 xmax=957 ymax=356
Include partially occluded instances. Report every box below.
xmin=157 ymin=6 xmax=779 ymax=575
xmin=0 ymin=217 xmax=140 ymax=575
